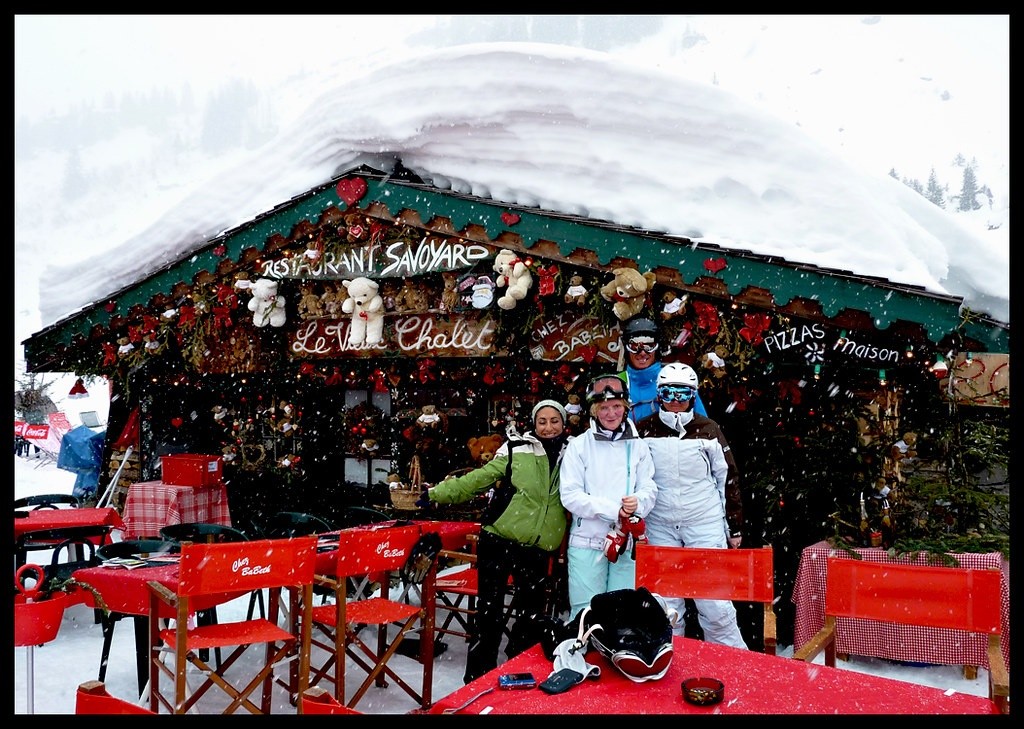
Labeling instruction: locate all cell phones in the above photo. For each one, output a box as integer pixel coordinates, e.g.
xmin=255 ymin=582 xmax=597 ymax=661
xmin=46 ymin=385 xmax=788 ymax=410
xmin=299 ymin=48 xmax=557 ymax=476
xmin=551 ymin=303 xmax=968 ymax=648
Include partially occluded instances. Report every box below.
xmin=539 ymin=669 xmax=583 ymax=694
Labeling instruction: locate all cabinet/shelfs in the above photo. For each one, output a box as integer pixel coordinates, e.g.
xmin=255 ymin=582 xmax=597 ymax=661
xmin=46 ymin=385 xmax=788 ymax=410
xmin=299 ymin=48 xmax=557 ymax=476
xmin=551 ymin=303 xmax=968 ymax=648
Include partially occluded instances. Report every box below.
xmin=243 ymin=390 xmax=296 ymax=472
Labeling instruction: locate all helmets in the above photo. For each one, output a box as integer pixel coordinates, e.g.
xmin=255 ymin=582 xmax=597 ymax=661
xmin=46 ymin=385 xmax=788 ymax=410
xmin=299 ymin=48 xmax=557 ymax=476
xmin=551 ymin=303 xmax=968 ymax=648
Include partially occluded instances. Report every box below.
xmin=622 ymin=318 xmax=661 ymax=340
xmin=657 ymin=363 xmax=699 ymax=389
xmin=586 ymin=585 xmax=674 ymax=680
xmin=587 ymin=374 xmax=631 ymax=403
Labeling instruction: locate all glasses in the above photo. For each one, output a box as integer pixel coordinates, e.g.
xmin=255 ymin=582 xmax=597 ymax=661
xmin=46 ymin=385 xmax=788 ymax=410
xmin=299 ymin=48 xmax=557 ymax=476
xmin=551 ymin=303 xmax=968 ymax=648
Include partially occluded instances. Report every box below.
xmin=625 ymin=339 xmax=659 ymax=354
xmin=657 ymin=386 xmax=696 ymax=403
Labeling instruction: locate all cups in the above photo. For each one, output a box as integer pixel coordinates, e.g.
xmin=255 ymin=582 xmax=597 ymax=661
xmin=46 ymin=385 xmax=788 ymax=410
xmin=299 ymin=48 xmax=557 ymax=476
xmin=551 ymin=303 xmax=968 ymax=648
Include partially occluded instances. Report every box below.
xmin=870 ymin=532 xmax=882 ymax=547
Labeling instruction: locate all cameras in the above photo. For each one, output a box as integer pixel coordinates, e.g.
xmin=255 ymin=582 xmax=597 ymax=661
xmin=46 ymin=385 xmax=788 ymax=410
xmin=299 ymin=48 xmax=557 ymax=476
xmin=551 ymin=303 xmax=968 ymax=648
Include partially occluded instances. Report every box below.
xmin=499 ymin=672 xmax=536 ymax=689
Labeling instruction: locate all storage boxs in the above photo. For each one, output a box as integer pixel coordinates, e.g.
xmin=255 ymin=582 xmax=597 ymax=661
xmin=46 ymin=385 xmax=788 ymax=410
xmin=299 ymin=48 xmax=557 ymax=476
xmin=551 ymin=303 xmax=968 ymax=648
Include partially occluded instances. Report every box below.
xmin=160 ymin=454 xmax=221 ymax=489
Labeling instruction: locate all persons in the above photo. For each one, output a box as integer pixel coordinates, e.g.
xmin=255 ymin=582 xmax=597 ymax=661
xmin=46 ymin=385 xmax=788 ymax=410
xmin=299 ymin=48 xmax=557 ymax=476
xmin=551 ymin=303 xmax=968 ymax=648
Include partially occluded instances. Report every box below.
xmin=595 ymin=317 xmax=709 ymax=423
xmin=561 ymin=374 xmax=660 ymax=626
xmin=414 ymin=399 xmax=580 ymax=688
xmin=637 ymin=364 xmax=751 ymax=650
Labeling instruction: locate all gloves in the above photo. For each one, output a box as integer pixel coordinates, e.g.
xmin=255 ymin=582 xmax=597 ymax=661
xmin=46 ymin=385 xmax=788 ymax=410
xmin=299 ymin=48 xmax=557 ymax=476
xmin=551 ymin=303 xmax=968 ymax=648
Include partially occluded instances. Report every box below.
xmin=416 ymin=491 xmax=432 ymax=509
xmin=629 ymin=515 xmax=649 ymax=560
xmin=602 ymin=511 xmax=629 ymax=564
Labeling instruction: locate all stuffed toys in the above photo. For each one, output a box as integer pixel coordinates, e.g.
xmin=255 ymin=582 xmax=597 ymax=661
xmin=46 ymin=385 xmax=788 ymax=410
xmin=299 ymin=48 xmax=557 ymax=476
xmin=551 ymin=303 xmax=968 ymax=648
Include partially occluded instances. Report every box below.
xmin=104 ymin=249 xmax=918 ymax=551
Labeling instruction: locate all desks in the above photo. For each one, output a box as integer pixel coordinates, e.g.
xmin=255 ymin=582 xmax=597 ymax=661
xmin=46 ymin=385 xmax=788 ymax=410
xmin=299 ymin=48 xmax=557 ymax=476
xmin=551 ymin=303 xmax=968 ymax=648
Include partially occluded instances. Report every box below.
xmin=411 ymin=632 xmax=1003 ymax=715
xmin=15 ymin=508 xmax=127 ymax=591
xmin=792 ymin=537 xmax=1009 ymax=680
xmin=118 ymin=481 xmax=231 ymax=540
xmin=71 ymin=519 xmax=481 ymax=702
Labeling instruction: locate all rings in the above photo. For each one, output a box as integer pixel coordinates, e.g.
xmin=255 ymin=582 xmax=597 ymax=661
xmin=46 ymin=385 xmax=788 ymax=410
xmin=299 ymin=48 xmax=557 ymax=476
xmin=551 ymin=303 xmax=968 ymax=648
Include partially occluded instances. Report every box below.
xmin=738 ymin=543 xmax=741 ymax=546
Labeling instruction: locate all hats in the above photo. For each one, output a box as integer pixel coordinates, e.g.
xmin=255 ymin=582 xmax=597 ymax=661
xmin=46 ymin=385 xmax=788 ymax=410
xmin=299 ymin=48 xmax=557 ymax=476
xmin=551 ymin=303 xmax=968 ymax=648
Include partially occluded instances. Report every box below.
xmin=533 ymin=399 xmax=567 ymax=426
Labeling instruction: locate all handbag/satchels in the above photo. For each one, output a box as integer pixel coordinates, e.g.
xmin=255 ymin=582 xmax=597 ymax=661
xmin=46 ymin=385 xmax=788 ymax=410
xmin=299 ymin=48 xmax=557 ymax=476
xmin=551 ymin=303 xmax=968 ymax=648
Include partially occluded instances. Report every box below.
xmin=478 ymin=440 xmax=515 ymax=526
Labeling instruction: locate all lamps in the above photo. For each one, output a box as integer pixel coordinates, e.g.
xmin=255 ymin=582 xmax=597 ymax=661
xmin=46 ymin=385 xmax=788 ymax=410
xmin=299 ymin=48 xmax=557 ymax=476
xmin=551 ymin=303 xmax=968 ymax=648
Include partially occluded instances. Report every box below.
xmin=932 ymin=361 xmax=948 ymax=379
xmin=68 ymin=377 xmax=90 ymax=400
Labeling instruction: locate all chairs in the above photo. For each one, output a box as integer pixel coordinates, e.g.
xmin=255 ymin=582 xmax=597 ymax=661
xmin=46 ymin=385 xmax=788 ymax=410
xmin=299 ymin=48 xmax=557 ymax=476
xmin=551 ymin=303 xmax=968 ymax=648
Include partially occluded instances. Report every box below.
xmin=13 ymin=495 xmax=1009 ymax=718
